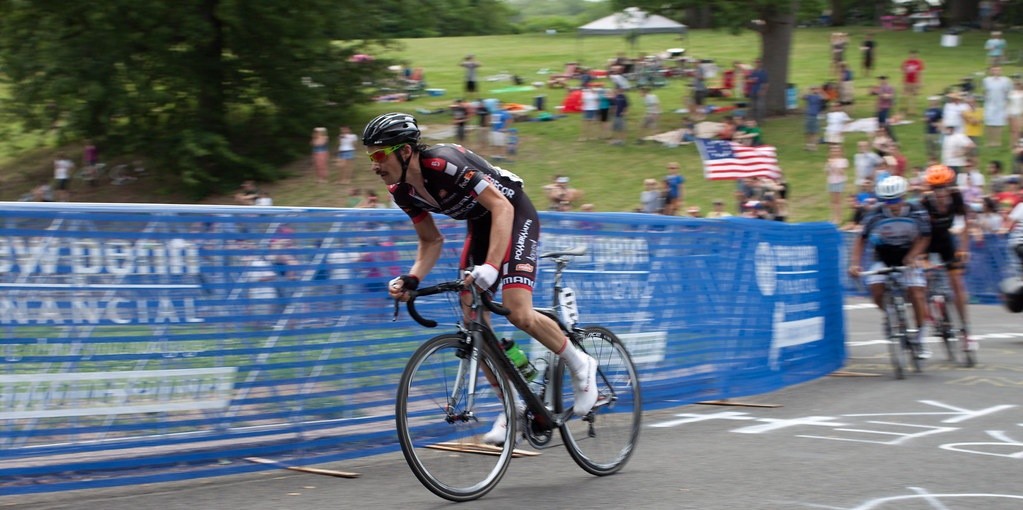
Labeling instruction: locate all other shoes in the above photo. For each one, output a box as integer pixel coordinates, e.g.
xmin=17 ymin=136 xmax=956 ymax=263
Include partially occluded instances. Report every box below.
xmin=573 ymin=354 xmax=597 ymax=416
xmin=484 ymin=415 xmax=521 ymax=443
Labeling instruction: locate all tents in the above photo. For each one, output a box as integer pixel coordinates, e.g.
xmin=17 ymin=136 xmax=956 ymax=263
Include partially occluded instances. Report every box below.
xmin=576 ymin=7 xmax=685 ymax=64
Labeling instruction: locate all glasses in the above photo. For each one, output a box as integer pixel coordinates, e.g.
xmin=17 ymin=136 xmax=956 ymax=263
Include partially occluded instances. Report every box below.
xmin=881 ymin=199 xmax=904 ymax=204
xmin=365 ymin=143 xmax=409 ymax=164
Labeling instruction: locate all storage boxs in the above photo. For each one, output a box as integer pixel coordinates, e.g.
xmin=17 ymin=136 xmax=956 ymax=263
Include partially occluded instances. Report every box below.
xmin=940 ymin=35 xmax=963 ymax=48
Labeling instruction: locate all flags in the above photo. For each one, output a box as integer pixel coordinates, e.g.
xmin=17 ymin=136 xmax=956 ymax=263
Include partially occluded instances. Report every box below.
xmin=699 ymin=137 xmax=781 ymax=180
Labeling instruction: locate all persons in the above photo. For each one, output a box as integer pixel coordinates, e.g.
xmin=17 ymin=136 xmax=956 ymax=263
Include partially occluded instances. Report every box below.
xmin=401 ymin=54 xmax=518 ymax=157
xmin=235 ymin=179 xmax=272 ymax=205
xmin=363 ymin=112 xmax=598 ymax=442
xmin=803 ymin=0 xmax=1023 ymax=292
xmin=542 ymin=53 xmax=789 ymax=221
xmin=848 ymin=164 xmax=978 ymax=360
xmin=341 ymin=188 xmax=399 ymax=209
xmin=33 ymin=141 xmax=98 ymax=201
xmin=311 ymin=126 xmax=358 ymax=184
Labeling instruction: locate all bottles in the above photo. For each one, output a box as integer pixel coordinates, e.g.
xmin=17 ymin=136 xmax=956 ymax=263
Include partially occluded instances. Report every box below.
xmin=500 ymin=337 xmax=539 ymax=383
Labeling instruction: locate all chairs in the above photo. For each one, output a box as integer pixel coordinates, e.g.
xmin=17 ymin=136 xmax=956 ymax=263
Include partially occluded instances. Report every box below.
xmin=546 ymin=60 xmax=576 ymax=87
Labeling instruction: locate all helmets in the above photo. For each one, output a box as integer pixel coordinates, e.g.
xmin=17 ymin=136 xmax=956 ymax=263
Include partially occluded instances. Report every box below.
xmin=361 ymin=112 xmax=421 ymax=148
xmin=924 ymin=165 xmax=955 ymax=184
xmin=874 ymin=175 xmax=910 ymax=198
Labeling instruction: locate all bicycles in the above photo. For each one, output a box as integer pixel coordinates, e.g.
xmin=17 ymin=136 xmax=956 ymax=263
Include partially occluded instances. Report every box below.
xmin=921 ymin=255 xmax=979 ymax=370
xmin=386 ymin=262 xmax=642 ymax=504
xmin=852 ymin=260 xmax=923 ymax=381
